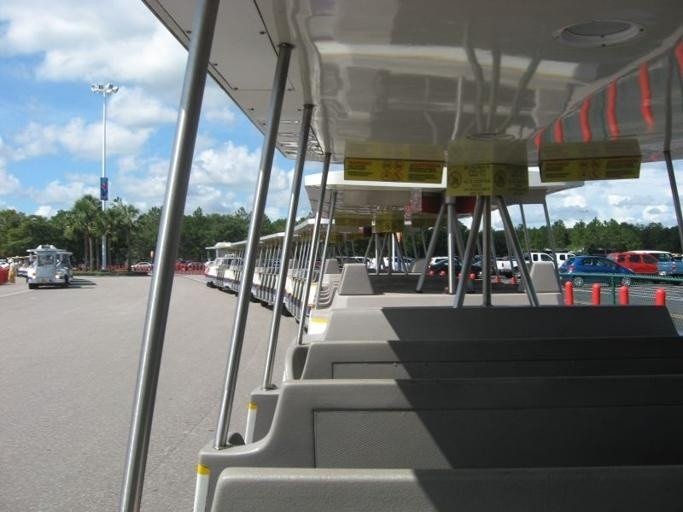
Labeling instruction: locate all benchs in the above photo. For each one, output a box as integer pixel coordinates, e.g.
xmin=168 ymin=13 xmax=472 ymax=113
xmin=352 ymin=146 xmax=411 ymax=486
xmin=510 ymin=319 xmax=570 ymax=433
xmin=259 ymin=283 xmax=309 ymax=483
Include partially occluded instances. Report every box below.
xmin=194 ymin=259 xmax=683 ymax=510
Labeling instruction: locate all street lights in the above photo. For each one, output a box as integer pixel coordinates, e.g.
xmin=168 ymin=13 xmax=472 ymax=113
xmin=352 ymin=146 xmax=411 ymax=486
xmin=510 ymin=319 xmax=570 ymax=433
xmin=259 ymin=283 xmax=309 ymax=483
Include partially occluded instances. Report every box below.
xmin=90 ymin=82 xmax=121 ymax=272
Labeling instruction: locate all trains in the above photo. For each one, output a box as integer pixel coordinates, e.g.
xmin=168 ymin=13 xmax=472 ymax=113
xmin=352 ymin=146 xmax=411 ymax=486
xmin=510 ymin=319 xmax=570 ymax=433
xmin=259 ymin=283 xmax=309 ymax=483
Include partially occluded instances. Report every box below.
xmin=0 ymin=244 xmax=75 ymax=290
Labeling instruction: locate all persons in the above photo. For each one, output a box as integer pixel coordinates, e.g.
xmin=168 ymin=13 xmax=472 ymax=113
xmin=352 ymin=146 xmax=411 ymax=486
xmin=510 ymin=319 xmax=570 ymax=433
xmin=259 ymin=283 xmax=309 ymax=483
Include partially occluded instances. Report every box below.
xmin=8 ymin=258 xmax=16 ymax=284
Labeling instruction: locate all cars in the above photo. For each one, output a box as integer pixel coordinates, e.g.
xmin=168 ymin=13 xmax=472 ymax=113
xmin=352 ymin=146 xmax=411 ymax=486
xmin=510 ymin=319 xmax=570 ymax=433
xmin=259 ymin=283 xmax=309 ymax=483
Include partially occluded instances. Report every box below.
xmin=131 ymin=261 xmax=153 ymax=272
